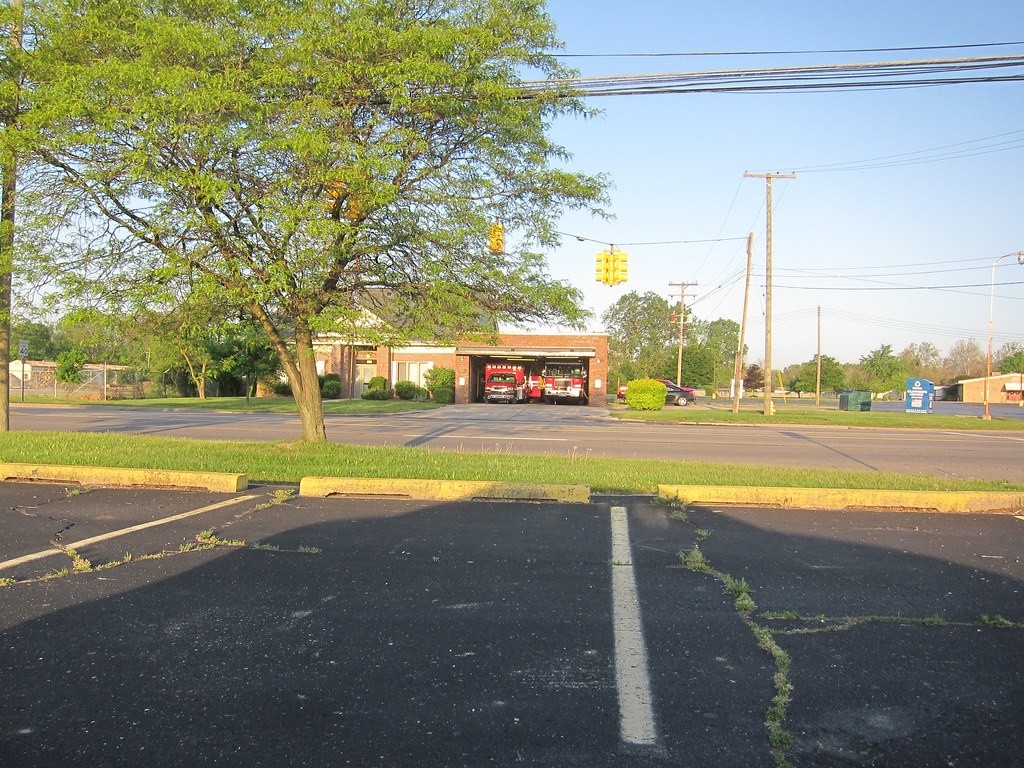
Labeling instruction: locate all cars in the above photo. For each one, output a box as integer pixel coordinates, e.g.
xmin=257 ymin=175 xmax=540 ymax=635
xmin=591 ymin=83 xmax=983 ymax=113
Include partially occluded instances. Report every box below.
xmin=618 ymin=378 xmax=695 ymax=402
xmin=664 ymin=385 xmax=696 ymax=406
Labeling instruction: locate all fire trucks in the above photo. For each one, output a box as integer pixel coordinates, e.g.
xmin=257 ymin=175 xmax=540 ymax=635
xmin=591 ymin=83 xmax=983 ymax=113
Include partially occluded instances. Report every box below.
xmin=483 ymin=361 xmax=542 ymax=404
xmin=543 ymin=358 xmax=587 ymax=405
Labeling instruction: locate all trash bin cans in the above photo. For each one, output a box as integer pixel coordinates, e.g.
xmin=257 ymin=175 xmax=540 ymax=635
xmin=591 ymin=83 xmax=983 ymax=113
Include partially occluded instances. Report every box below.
xmin=839 ymin=390 xmax=872 ymax=412
